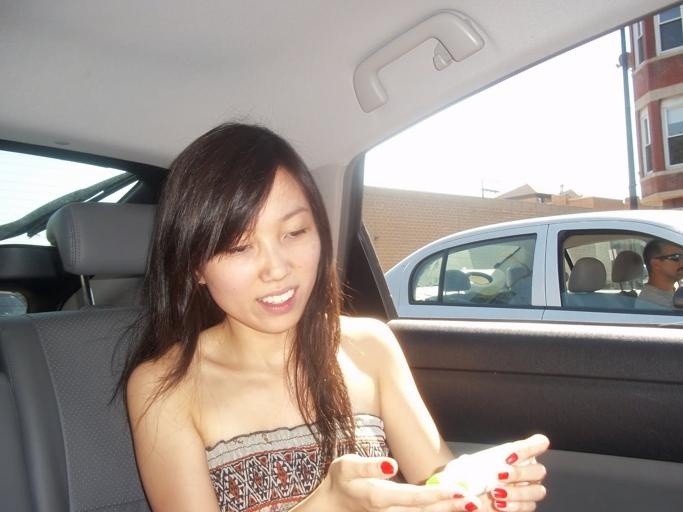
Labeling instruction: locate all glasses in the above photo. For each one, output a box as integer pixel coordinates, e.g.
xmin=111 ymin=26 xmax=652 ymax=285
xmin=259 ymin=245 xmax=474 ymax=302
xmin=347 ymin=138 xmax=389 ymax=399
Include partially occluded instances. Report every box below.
xmin=653 ymin=253 xmax=682 ymax=263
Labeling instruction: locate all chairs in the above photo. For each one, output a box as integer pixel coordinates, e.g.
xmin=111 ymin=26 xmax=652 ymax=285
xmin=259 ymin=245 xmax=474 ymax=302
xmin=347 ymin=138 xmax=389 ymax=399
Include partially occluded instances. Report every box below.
xmin=611 ymin=250 xmax=647 ymax=305
xmin=2 ymin=200 xmax=302 ymax=512
xmin=439 ymin=263 xmax=529 ymax=306
xmin=568 ymin=258 xmax=610 ymax=307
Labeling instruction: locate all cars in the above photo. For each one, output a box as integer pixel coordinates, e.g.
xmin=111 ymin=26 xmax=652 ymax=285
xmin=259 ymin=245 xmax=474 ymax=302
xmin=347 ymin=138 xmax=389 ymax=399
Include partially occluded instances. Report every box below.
xmin=382 ymin=205 xmax=682 ymax=329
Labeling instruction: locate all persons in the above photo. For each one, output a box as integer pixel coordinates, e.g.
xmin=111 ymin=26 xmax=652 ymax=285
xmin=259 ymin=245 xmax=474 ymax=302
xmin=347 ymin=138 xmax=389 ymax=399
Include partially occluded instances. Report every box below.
xmin=628 ymin=236 xmax=682 ymax=312
xmin=106 ymin=118 xmax=551 ymax=512
